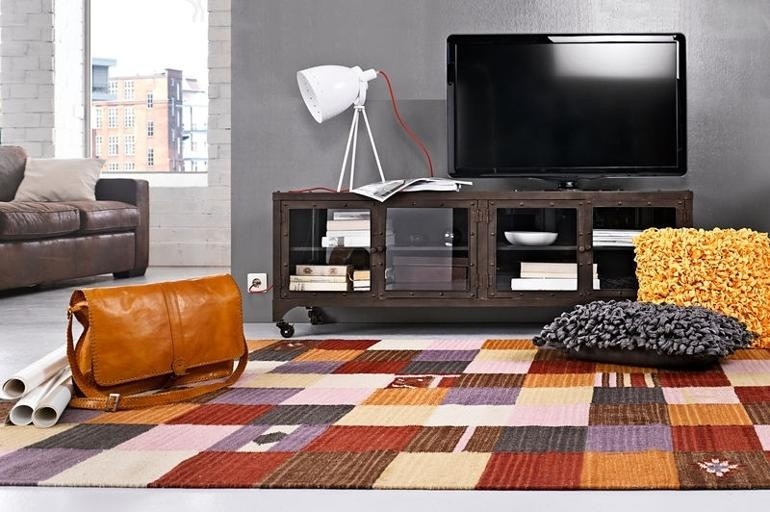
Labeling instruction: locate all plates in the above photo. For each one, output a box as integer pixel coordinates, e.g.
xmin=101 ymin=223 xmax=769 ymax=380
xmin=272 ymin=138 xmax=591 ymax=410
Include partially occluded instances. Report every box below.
xmin=505 ymin=231 xmax=558 ymax=246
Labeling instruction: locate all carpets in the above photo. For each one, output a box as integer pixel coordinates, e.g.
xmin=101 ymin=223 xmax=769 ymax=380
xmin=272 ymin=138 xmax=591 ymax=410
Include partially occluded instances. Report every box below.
xmin=0 ymin=337 xmax=769 ymax=489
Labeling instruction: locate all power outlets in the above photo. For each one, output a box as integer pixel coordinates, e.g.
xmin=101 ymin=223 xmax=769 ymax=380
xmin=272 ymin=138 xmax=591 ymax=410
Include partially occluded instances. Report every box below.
xmin=247 ymin=272 xmax=268 ymax=293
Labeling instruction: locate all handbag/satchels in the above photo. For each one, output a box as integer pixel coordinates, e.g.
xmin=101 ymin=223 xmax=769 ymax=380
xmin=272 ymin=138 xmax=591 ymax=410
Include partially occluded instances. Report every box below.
xmin=65 ymin=274 xmax=248 ymax=413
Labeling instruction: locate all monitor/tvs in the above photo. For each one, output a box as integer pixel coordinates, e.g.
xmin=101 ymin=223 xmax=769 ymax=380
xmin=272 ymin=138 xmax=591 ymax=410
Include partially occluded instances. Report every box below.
xmin=447 ymin=31 xmax=688 ymax=192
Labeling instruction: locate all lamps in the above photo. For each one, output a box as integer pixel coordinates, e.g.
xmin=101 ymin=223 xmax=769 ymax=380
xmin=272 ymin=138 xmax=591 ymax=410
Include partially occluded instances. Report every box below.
xmin=294 ymin=64 xmax=386 ymax=195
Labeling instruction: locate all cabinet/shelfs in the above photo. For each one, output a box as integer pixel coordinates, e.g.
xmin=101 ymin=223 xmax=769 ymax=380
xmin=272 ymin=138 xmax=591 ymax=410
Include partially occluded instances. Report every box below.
xmin=271 ymin=189 xmax=693 ymax=341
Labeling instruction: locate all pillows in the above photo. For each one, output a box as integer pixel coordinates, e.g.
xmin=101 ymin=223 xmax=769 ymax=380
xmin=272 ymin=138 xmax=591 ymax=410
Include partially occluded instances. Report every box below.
xmin=12 ymin=156 xmax=106 ymax=204
xmin=532 ymin=300 xmax=756 ymax=371
xmin=631 ymin=227 xmax=770 ymax=349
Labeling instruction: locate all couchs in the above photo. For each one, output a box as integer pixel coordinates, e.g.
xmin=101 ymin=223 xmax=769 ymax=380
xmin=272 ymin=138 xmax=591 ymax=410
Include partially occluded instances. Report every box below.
xmin=1 ymin=146 xmax=149 ymax=295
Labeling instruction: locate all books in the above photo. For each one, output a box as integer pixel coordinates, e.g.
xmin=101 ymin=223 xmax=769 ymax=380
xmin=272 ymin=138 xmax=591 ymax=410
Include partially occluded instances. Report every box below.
xmin=321 ymin=210 xmax=395 ymax=248
xmin=593 ymin=229 xmax=644 ymax=247
xmin=511 ymin=261 xmax=601 ymax=291
xmin=288 ymin=263 xmax=371 ymax=291
